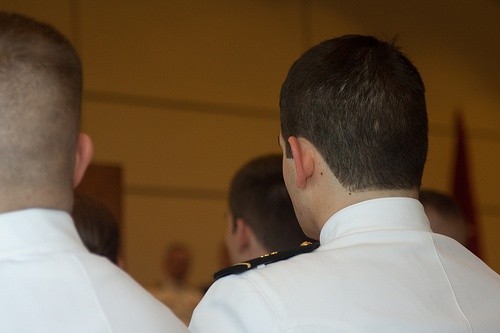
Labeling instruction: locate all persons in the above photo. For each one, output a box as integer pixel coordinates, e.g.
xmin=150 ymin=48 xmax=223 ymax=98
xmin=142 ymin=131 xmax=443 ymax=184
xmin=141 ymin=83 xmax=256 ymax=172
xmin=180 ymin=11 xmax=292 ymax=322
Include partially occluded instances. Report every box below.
xmin=0 ymin=11 xmax=190 ymax=333
xmin=72 ymin=34 xmax=499 ymax=333
